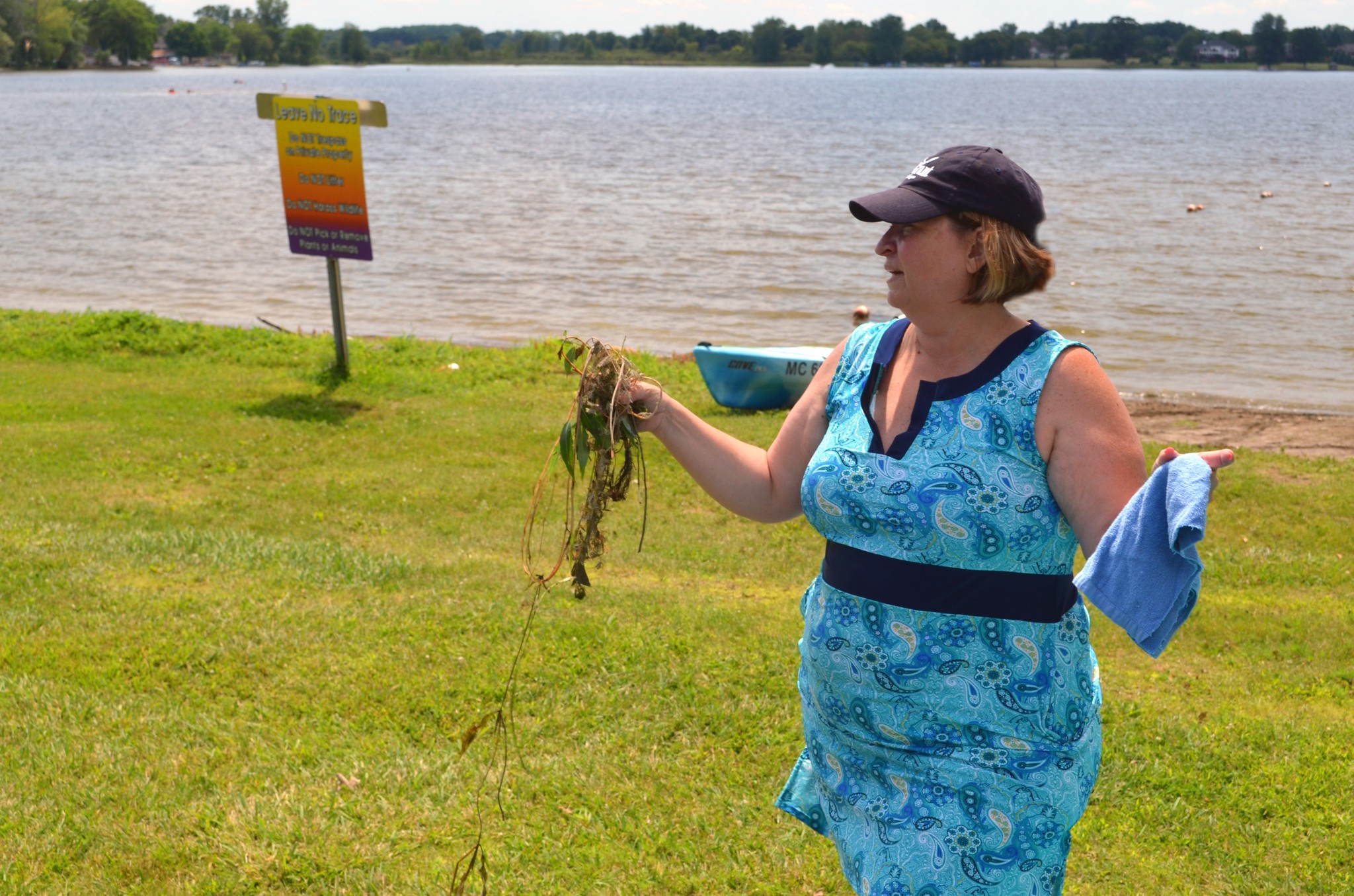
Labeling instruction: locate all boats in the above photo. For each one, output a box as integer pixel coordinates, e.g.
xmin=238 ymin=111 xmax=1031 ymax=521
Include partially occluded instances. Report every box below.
xmin=694 ymin=345 xmax=836 ymax=409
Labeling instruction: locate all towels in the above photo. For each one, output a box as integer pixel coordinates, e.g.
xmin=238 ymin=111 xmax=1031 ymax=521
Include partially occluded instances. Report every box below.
xmin=1071 ymin=453 xmax=1212 ymax=658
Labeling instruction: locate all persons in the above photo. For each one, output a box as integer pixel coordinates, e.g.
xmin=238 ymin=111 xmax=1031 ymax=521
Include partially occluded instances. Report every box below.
xmin=586 ymin=144 xmax=1234 ymax=896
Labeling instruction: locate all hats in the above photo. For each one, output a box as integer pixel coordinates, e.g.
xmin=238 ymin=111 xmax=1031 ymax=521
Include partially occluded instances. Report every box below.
xmin=849 ymin=146 xmax=1046 ymax=237
